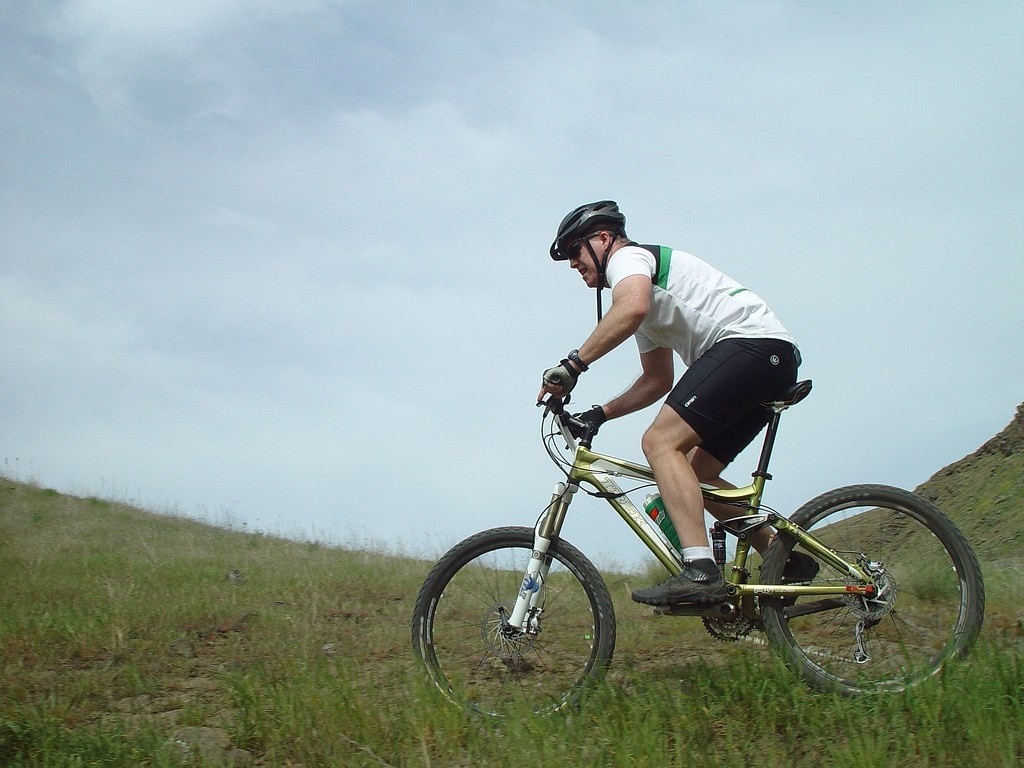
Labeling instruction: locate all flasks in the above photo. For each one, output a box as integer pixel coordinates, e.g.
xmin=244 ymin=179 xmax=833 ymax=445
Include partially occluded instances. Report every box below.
xmin=643 ymin=493 xmax=683 ymax=556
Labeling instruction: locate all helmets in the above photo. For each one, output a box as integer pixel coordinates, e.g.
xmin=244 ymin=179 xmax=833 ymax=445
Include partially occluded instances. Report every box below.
xmin=549 ymin=201 xmax=625 ymax=261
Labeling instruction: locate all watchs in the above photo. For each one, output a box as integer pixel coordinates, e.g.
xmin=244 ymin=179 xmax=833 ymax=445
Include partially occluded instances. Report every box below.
xmin=568 ymin=349 xmax=589 ymax=372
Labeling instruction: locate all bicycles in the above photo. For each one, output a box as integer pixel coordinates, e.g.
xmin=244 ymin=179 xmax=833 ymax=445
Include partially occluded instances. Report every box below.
xmin=412 ymin=374 xmax=985 ymax=725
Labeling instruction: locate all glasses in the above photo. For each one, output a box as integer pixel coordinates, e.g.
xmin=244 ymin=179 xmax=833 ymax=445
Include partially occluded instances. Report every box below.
xmin=568 ymin=232 xmax=599 ymax=261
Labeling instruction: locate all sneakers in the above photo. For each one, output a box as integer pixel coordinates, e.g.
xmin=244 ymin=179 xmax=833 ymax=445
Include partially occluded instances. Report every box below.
xmin=777 ymin=551 xmax=820 ymax=626
xmin=630 ymin=562 xmax=727 ymax=605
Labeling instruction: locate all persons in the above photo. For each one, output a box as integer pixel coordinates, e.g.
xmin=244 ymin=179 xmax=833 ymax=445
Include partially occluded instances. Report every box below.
xmin=537 ymin=200 xmax=802 ymax=606
